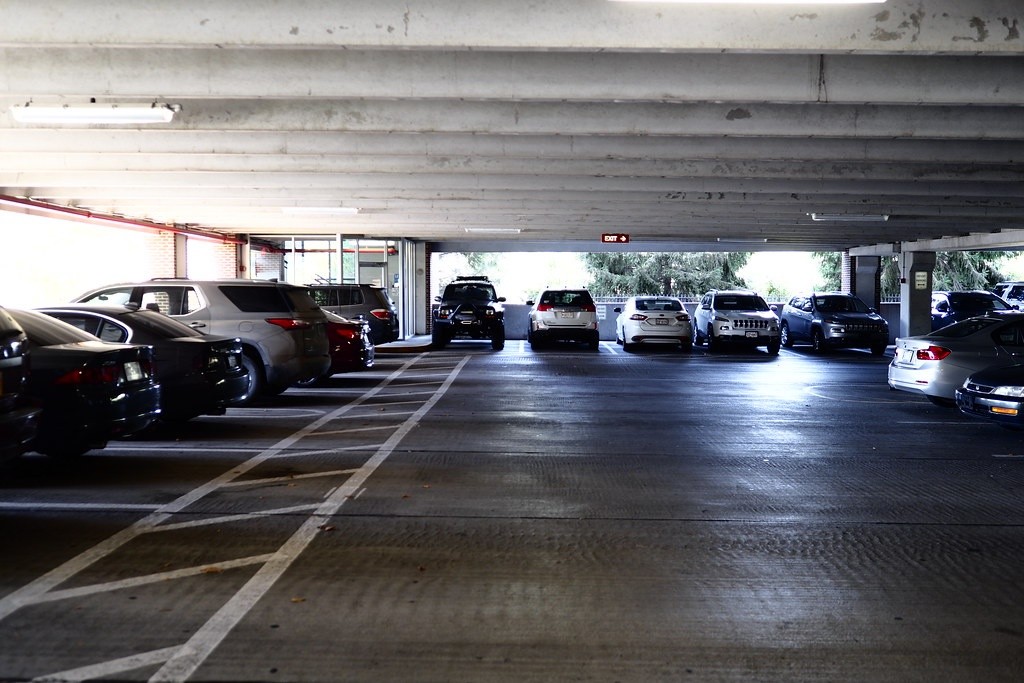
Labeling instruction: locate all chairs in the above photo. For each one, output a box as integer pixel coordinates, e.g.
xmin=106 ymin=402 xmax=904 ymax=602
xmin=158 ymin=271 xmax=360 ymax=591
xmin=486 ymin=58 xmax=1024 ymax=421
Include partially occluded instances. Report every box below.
xmin=664 ymin=305 xmax=672 ymax=311
xmin=146 ymin=303 xmax=159 ymax=314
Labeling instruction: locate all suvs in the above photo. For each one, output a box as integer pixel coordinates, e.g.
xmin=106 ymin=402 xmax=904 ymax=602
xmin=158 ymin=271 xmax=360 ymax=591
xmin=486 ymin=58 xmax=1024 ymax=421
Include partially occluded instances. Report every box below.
xmin=694 ymin=289 xmax=780 ymax=354
xmin=526 ymin=288 xmax=599 ymax=350
xmin=992 ymin=281 xmax=1024 ymax=310
xmin=71 ymin=277 xmax=332 ymax=402
xmin=299 ymin=283 xmax=399 ymax=346
xmin=433 ymin=275 xmax=505 ymax=350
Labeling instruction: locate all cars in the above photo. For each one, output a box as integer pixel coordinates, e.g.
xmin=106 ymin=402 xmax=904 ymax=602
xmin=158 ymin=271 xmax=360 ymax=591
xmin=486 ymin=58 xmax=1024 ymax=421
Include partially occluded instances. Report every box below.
xmin=0 ymin=304 xmax=161 ymax=455
xmin=954 ymin=362 xmax=1024 ymax=427
xmin=294 ymin=305 xmax=374 ymax=386
xmin=886 ymin=310 xmax=1024 ymax=406
xmin=613 ymin=296 xmax=694 ymax=351
xmin=931 ymin=290 xmax=1020 ymax=332
xmin=31 ymin=307 xmax=252 ymax=424
xmin=780 ymin=291 xmax=889 ymax=355
